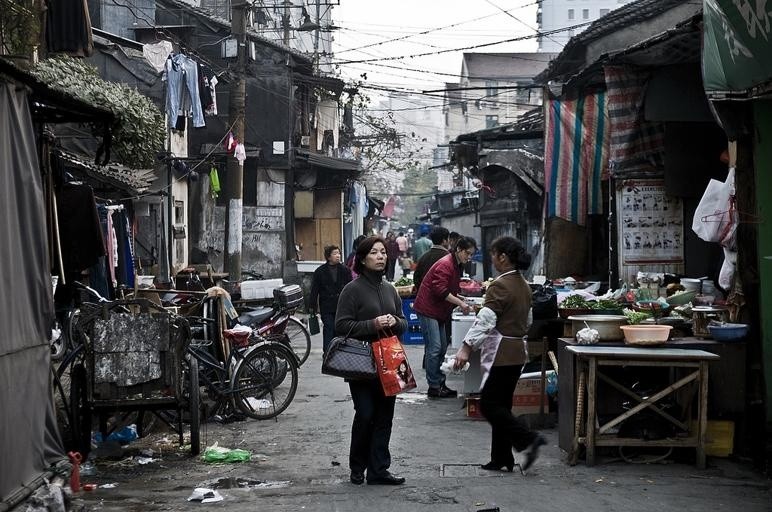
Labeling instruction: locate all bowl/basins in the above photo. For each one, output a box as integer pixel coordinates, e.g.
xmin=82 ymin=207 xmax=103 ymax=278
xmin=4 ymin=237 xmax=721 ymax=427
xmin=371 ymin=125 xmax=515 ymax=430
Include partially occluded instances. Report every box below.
xmin=634 ymin=277 xmax=715 ymax=316
xmin=708 ymin=323 xmax=749 ymax=339
xmin=557 ymin=307 xmax=618 ymax=320
xmin=568 ymin=314 xmax=628 ymax=339
xmin=620 ymin=325 xmax=673 ymax=343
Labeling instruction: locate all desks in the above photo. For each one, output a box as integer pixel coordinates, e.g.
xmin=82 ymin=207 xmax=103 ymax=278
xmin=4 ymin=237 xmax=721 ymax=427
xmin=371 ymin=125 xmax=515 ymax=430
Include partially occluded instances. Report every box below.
xmin=565 ymin=345 xmax=721 ymax=479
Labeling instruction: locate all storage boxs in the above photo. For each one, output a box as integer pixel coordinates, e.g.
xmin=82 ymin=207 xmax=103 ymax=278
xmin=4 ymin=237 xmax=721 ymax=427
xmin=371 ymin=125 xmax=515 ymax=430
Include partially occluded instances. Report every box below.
xmin=451 ymin=312 xmax=476 ymax=349
xmin=511 ymin=377 xmax=551 ymax=421
xmin=462 ymin=395 xmax=486 ymax=418
xmin=240 ymin=279 xmax=284 ymax=300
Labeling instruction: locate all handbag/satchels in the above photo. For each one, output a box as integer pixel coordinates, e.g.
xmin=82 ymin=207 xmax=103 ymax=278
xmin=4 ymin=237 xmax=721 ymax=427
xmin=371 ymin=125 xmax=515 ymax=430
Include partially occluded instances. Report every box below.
xmin=307 ymin=311 xmax=321 ymax=336
xmin=370 ymin=320 xmax=419 ymax=398
xmin=319 ymin=318 xmax=380 ymax=383
xmin=689 ymin=165 xmax=740 ymax=247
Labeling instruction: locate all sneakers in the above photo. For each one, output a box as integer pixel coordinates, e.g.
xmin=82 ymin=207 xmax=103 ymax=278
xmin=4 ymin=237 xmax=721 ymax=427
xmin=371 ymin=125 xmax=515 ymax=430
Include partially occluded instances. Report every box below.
xmin=427 ymin=385 xmax=459 ymax=400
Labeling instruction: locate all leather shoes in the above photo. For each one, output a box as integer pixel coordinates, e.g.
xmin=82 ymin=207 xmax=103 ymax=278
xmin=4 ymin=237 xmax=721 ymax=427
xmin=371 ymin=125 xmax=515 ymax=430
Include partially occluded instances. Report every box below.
xmin=366 ymin=470 xmax=406 ymax=485
xmin=349 ymin=469 xmax=365 ymax=484
xmin=518 ymin=434 xmax=548 ymax=477
xmin=480 ymin=450 xmax=515 ymax=474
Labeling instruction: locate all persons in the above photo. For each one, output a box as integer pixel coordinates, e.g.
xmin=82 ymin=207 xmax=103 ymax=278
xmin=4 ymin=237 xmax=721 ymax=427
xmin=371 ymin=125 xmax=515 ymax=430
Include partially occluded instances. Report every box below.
xmin=395 ymin=358 xmax=410 ymax=383
xmin=451 ymin=234 xmax=549 ymax=473
xmin=337 ymin=237 xmax=407 ymax=486
xmin=346 ymin=231 xmax=466 ymax=281
xmin=410 ymin=235 xmax=479 ymax=399
xmin=306 ymin=244 xmax=354 ymax=360
xmin=413 ymin=227 xmax=451 ymax=369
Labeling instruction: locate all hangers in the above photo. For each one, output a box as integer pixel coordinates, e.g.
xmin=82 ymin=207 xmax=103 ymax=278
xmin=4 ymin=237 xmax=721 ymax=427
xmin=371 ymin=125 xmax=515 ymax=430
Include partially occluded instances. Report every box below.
xmin=148 ymin=31 xmax=161 ymax=43
xmin=701 ymin=196 xmax=765 ymax=224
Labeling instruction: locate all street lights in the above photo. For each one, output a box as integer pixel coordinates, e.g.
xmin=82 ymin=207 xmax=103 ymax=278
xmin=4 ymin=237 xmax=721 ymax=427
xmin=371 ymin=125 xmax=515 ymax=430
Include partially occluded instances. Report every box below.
xmin=220 ymin=3 xmax=322 ymax=293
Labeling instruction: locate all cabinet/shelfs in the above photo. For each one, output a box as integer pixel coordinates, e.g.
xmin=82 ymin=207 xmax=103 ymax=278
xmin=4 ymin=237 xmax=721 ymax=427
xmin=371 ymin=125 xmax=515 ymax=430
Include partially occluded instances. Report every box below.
xmin=557 ymin=338 xmax=747 ymax=459
